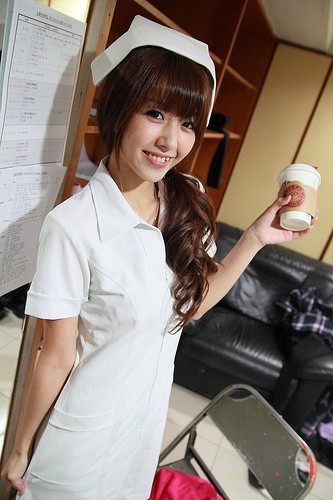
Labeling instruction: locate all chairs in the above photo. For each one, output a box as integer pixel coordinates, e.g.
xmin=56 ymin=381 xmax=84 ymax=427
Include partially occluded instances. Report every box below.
xmin=155 ymin=384 xmax=316 ymax=500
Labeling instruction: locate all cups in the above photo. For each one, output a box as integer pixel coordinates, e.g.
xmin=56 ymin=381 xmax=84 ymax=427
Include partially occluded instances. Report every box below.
xmin=276 ymin=163 xmax=321 ymax=231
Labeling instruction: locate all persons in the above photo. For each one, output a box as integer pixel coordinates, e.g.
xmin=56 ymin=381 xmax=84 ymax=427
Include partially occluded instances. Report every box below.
xmin=0 ymin=15 xmax=319 ymax=500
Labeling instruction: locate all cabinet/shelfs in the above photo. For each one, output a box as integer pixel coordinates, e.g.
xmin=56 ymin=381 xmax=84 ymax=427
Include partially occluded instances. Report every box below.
xmin=54 ymin=0 xmax=280 ymax=226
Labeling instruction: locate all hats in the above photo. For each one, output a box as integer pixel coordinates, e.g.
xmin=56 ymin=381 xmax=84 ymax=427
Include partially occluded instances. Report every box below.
xmin=90 ymin=14 xmax=216 ymax=128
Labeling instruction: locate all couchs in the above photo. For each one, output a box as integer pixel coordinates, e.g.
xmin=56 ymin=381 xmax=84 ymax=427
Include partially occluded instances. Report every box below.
xmin=176 ymin=219 xmax=333 ymax=433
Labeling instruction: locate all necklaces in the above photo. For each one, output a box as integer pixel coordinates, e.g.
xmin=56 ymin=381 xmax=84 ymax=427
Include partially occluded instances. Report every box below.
xmin=152 ymin=187 xmax=159 ymax=227
